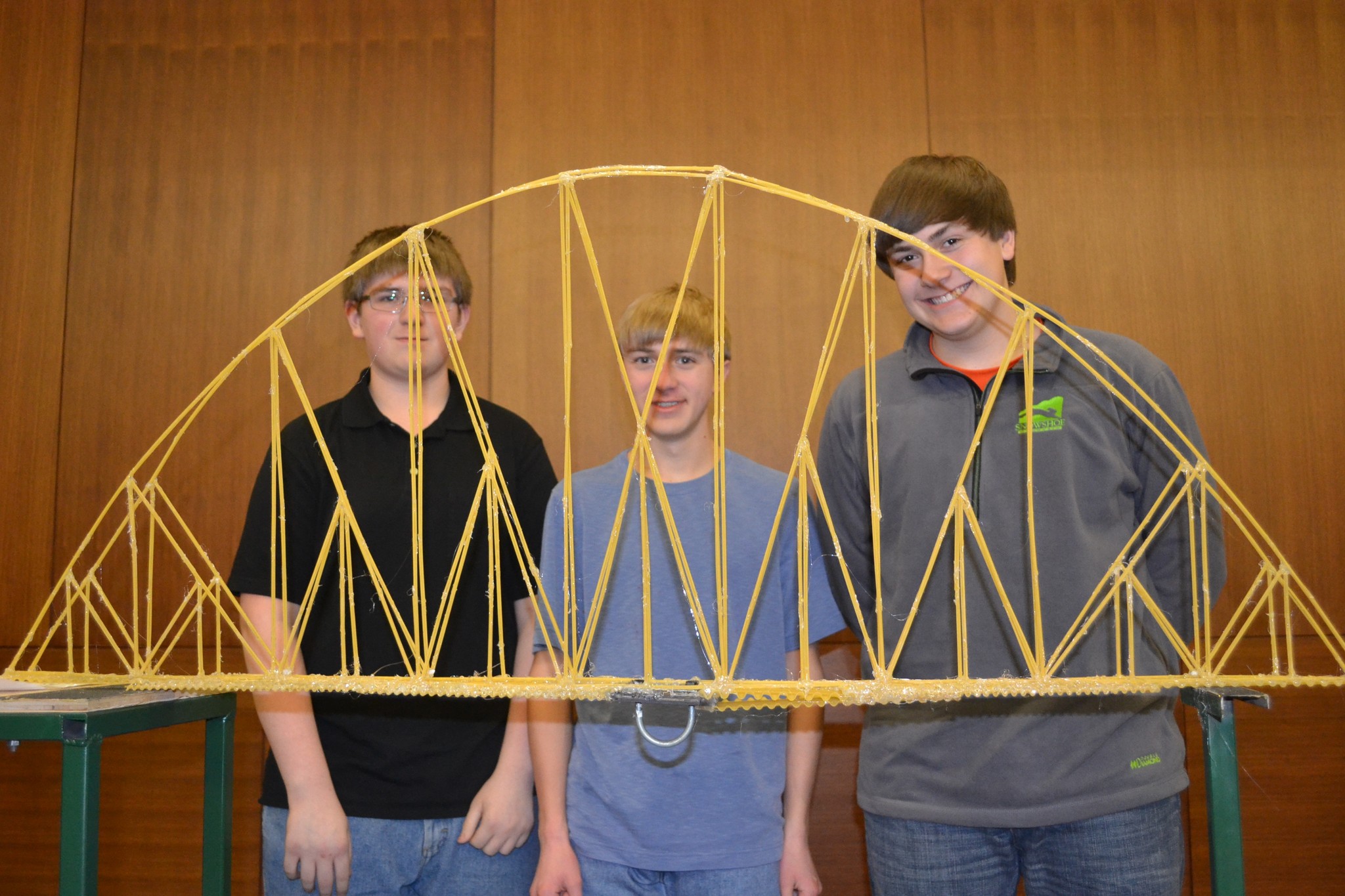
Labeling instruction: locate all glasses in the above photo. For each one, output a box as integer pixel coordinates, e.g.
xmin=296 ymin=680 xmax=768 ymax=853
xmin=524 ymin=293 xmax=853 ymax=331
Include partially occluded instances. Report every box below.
xmin=355 ymin=288 xmax=465 ymax=313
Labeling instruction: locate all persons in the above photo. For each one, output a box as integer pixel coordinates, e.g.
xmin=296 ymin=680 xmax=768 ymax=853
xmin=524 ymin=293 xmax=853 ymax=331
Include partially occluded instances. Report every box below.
xmin=221 ymin=224 xmax=557 ymax=896
xmin=818 ymin=156 xmax=1230 ymax=896
xmin=528 ymin=287 xmax=850 ymax=896
